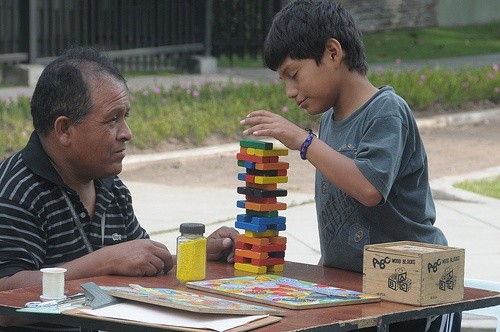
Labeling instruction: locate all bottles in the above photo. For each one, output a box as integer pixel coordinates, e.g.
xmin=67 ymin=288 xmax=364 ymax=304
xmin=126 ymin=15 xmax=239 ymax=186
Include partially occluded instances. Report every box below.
xmin=176 ymin=223 xmax=207 ymax=284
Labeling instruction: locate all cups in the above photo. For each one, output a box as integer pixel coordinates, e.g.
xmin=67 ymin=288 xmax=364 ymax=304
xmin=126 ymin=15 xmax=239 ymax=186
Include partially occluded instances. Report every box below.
xmin=40 ymin=268 xmax=68 ymax=297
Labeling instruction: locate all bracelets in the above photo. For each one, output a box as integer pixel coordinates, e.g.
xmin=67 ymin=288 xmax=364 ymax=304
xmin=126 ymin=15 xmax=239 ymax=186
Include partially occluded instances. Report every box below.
xmin=300 ymin=127 xmax=317 ymax=160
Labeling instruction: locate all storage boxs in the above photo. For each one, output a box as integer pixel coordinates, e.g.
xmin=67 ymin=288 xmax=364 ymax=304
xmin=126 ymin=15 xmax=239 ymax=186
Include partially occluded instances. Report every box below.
xmin=361 ymin=241 xmax=465 ymax=307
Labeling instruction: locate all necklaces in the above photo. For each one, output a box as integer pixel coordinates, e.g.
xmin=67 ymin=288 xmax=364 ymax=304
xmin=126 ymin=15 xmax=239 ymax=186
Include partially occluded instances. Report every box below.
xmin=61 ymin=189 xmax=105 ymax=254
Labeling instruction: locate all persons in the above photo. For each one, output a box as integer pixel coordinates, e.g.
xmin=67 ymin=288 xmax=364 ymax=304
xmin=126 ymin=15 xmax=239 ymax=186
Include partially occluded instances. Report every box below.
xmin=240 ymin=1 xmax=462 ymax=332
xmin=1 ymin=41 xmax=239 ymax=332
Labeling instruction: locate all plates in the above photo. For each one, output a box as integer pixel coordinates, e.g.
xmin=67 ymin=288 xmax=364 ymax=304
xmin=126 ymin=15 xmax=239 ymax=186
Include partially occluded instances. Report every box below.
xmin=39 ymin=295 xmax=67 ymax=301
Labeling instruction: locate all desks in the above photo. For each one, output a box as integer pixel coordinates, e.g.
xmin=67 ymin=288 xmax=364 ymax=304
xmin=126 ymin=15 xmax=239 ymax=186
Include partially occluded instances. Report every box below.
xmin=0 ymin=260 xmax=500 ymax=332
